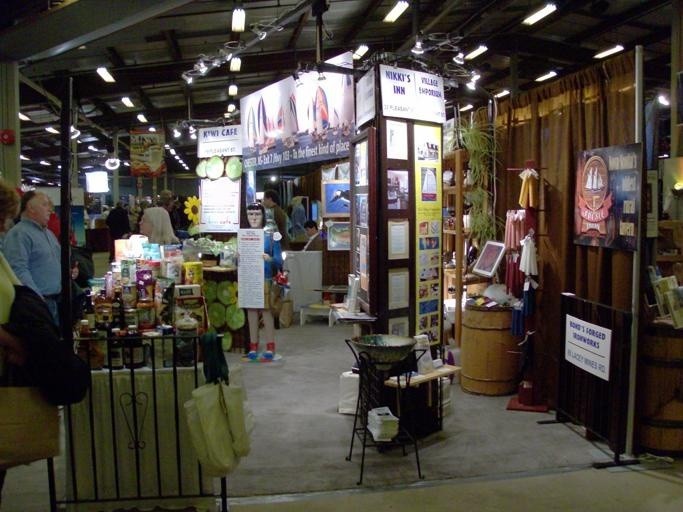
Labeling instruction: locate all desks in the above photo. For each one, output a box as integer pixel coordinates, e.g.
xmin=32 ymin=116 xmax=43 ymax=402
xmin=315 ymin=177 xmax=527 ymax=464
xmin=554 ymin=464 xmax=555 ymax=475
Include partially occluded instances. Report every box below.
xmin=66 ymin=361 xmax=217 ymax=511
xmin=381 ymin=362 xmax=464 ymax=442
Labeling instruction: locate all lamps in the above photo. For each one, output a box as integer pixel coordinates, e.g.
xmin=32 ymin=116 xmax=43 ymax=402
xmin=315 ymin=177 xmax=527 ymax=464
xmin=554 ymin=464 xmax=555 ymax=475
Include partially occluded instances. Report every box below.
xmin=94 ymin=67 xmax=190 ymax=170
xmin=592 ymin=45 xmax=625 ymax=60
xmin=520 ymin=4 xmax=559 ymax=25
xmin=224 ymin=0 xmax=247 ymax=121
xmin=352 ymin=44 xmax=369 ymax=61
xmin=382 ymin=0 xmax=412 ymax=24
xmin=457 ymin=71 xmax=559 ymax=111
xmin=461 ymin=45 xmax=490 ymax=61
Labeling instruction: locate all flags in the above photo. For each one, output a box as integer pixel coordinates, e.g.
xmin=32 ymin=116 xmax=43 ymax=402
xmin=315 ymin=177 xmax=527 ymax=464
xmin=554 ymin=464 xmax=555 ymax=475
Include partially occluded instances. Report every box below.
xmin=126 ymin=128 xmax=165 ymax=180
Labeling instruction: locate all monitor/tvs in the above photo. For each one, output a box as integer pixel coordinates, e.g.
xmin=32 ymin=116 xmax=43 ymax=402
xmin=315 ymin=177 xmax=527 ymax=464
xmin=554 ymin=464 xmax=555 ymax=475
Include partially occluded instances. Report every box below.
xmin=472 ymin=241 xmax=506 ymax=278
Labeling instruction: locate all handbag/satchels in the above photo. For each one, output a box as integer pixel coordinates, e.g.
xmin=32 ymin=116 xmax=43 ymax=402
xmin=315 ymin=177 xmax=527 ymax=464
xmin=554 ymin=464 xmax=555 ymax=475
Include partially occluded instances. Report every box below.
xmin=185 ymin=365 xmax=251 ymax=476
xmin=0 ymin=386 xmax=60 ymax=468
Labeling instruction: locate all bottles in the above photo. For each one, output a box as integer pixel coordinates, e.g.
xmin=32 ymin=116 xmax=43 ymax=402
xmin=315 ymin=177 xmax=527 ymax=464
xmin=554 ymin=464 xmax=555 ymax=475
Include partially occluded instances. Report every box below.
xmin=83 ymin=257 xmax=158 ymax=336
xmin=219 ymin=242 xmax=235 ymax=268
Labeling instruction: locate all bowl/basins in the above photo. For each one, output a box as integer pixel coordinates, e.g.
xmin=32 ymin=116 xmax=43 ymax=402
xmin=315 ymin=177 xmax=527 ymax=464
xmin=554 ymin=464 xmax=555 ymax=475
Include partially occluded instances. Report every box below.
xmin=347 ymin=333 xmax=417 ymax=371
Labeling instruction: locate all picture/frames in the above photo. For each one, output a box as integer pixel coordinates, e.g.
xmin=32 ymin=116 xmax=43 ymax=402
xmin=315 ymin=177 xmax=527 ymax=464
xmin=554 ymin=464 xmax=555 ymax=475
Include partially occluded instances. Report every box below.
xmin=321 ymin=178 xmax=350 ymax=219
xmin=326 ymin=221 xmax=351 ymax=252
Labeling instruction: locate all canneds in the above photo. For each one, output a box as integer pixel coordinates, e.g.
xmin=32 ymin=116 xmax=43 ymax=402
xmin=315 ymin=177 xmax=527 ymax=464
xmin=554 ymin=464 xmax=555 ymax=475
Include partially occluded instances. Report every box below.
xmin=93 ymin=261 xmax=161 ymax=331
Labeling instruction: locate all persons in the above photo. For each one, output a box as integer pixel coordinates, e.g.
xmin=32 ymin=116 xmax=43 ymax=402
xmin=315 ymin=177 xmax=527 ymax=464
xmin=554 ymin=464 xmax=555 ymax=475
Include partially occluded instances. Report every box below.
xmin=299 ymin=221 xmax=322 ymax=252
xmin=262 ymin=190 xmax=291 ymax=252
xmin=234 ymin=201 xmax=286 ymax=363
xmin=0 ymin=179 xmax=93 ymax=494
xmin=5 ymin=191 xmax=79 ymax=336
xmin=95 ymin=190 xmax=189 ymax=250
